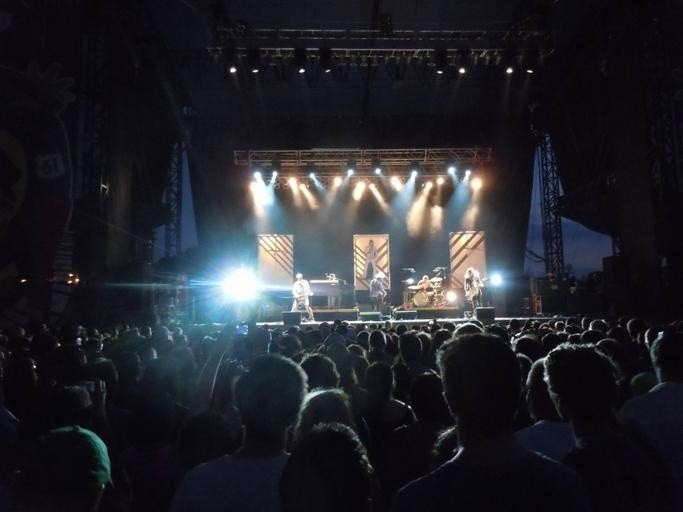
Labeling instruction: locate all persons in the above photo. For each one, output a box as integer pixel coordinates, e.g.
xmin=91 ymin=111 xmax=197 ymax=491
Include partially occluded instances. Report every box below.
xmin=0 ymin=267 xmax=683 ymax=512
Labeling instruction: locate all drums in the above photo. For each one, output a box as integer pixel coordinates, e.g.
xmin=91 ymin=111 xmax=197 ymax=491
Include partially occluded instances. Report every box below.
xmin=413 ymin=292 xmax=434 ymax=307
xmin=433 ymin=286 xmax=443 ymax=307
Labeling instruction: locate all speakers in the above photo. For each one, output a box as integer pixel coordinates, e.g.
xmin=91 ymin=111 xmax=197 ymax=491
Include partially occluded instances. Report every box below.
xmin=282 ymin=311 xmax=302 ymax=323
xmin=393 ymin=311 xmax=417 ymax=320
xmin=474 ymin=306 xmax=496 ymax=320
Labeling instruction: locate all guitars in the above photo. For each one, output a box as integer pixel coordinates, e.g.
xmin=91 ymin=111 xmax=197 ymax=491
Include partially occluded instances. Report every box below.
xmin=465 ymin=277 xmax=488 ymax=302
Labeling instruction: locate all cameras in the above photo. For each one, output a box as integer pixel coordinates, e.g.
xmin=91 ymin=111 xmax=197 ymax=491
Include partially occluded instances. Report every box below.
xmin=235 ymin=322 xmax=249 ymax=336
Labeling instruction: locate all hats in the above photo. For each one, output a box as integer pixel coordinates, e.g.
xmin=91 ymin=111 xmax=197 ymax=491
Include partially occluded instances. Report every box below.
xmin=46 ymin=426 xmax=115 ymax=488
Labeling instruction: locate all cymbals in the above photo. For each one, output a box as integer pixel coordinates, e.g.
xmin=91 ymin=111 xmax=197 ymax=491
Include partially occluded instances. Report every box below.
xmin=408 ymin=286 xmax=422 ymax=290
xmin=431 ymin=278 xmax=443 ymax=282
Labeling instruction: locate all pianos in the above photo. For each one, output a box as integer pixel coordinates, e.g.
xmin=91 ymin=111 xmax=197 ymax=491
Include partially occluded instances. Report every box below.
xmin=309 ymin=279 xmax=342 ymax=310
